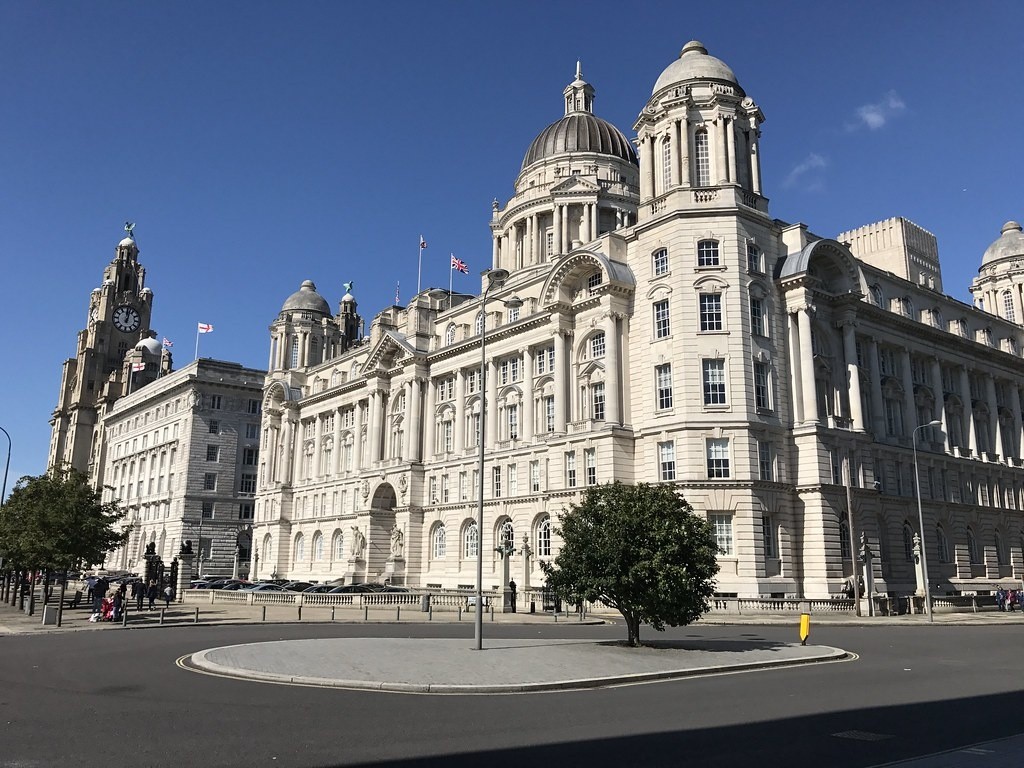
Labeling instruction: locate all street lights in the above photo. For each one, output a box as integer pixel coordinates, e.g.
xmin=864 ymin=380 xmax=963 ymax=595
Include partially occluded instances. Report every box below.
xmin=912 ymin=419 xmax=943 ymax=620
xmin=474 ymin=267 xmax=524 ymax=651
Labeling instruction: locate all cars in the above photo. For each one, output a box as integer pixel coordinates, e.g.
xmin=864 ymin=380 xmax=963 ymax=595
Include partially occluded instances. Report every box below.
xmin=188 ymin=577 xmax=409 ymax=603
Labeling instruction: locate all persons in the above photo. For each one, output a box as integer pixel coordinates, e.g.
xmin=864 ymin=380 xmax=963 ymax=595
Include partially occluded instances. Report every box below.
xmin=164 ymin=584 xmax=174 ymax=609
xmin=390 ymin=523 xmax=401 ymax=557
xmin=1016 ymin=589 xmax=1024 ymax=613
xmin=351 ymin=525 xmax=364 ymax=556
xmin=1007 ymin=588 xmax=1015 ymax=612
xmin=133 ymin=578 xmax=146 ymax=611
xmin=147 ymin=579 xmax=159 ymax=611
xmin=996 ymin=586 xmax=1006 ymax=612
xmin=70 ymin=590 xmax=82 ymax=608
xmin=86 ymin=577 xmax=127 ymax=621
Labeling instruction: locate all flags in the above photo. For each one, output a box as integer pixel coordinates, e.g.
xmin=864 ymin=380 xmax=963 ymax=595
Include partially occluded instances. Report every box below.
xmin=132 ymin=362 xmax=146 ymax=372
xmin=451 ymin=255 xmax=469 ymax=275
xmin=197 ymin=322 xmax=214 ymax=333
xmin=163 ymin=338 xmax=173 ymax=347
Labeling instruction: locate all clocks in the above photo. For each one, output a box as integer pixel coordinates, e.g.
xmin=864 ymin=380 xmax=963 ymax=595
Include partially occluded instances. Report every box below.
xmin=112 ymin=306 xmax=141 ymax=333
